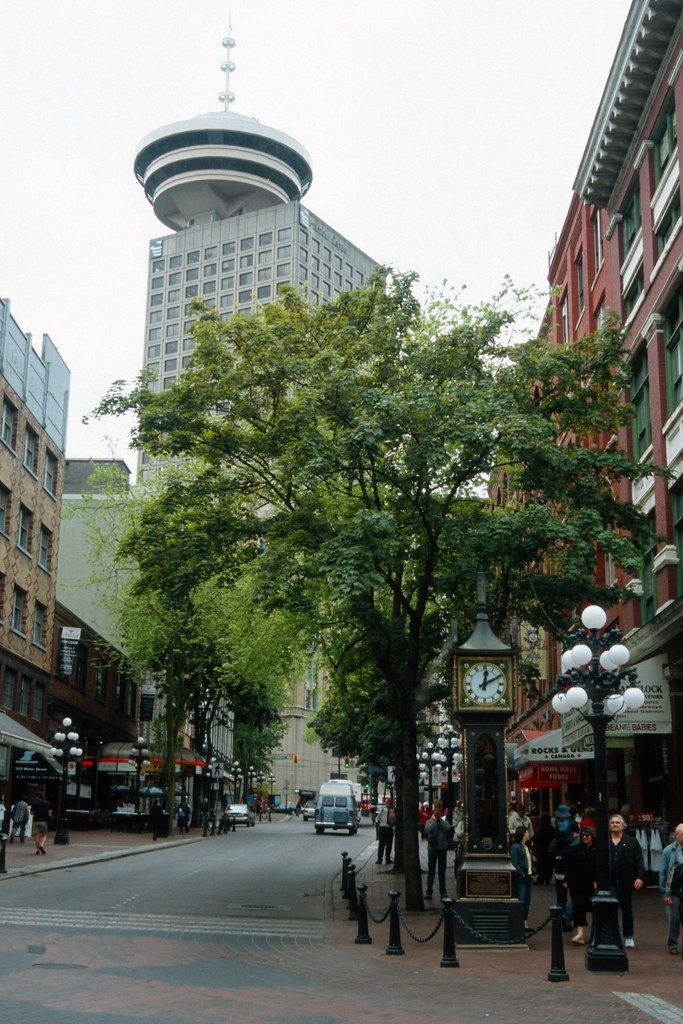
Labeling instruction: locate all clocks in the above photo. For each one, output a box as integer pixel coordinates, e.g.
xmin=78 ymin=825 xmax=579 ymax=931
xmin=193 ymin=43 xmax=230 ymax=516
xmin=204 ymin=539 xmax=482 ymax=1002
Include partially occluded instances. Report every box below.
xmin=462 ymin=662 xmax=506 ymax=708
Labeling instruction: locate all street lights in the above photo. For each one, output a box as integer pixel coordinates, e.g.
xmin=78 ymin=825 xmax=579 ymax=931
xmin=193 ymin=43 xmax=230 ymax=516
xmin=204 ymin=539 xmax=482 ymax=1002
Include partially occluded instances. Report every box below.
xmin=416 ymin=742 xmax=443 ymax=810
xmin=433 ymin=724 xmax=462 ymax=849
xmin=267 ymin=772 xmax=277 ymax=813
xmin=257 ymin=770 xmax=265 ymax=814
xmin=48 ymin=717 xmax=84 ymax=845
xmin=205 ymin=756 xmax=217 ymax=821
xmin=551 ymin=605 xmax=646 ymax=973
xmin=127 ymin=736 xmax=152 ymax=814
xmin=229 ymin=761 xmax=243 ymax=804
xmin=247 ymin=766 xmax=257 ymax=812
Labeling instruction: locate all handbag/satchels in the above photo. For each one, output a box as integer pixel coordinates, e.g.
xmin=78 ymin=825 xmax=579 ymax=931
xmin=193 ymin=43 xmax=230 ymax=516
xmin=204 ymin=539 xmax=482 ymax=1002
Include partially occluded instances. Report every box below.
xmin=179 ymin=808 xmax=184 ymax=817
xmin=428 ymin=837 xmax=438 ymax=847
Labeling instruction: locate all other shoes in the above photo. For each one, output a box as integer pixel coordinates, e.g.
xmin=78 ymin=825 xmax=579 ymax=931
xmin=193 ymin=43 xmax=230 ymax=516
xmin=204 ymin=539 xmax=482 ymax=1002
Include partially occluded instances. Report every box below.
xmin=625 ymin=938 xmax=635 ymax=948
xmin=525 ymin=928 xmax=534 ymax=932
xmin=38 ymin=846 xmax=46 ymax=854
xmin=386 ymin=860 xmax=393 ymax=864
xmin=441 ymin=893 xmax=447 ymax=899
xmin=375 ymin=860 xmax=382 ymax=864
xmin=670 ymin=947 xmax=678 ymax=954
xmin=424 ymin=893 xmax=432 ymax=899
xmin=36 ymin=850 xmax=40 ymax=854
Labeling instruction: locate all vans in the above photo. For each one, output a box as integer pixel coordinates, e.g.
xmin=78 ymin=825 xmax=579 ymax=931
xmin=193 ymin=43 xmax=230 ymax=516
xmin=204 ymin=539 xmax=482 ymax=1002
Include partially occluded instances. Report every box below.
xmin=314 ymin=779 xmax=362 ymax=836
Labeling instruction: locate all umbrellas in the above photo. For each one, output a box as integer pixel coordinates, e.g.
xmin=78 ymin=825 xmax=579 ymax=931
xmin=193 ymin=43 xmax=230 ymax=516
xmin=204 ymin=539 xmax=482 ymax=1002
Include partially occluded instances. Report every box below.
xmin=106 ymin=781 xmax=131 ymax=794
xmin=173 ymin=786 xmax=190 ymax=796
xmin=138 ymin=784 xmax=167 ymax=797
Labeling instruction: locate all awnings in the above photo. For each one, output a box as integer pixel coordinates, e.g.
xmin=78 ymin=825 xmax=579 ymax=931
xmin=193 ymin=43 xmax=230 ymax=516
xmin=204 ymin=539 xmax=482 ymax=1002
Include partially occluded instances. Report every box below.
xmin=9 ymin=746 xmax=63 ymax=781
xmin=301 ymin=790 xmax=314 ymax=795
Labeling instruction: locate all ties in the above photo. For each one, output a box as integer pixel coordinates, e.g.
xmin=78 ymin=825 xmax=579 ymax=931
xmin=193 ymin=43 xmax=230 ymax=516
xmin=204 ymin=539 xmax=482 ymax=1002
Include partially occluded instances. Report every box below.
xmin=387 ymin=811 xmax=390 ymax=824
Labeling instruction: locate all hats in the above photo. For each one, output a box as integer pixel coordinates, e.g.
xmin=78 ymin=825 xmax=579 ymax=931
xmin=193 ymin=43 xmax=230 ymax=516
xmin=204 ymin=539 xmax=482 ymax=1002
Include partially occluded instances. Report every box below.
xmin=555 ymin=805 xmax=571 ymax=817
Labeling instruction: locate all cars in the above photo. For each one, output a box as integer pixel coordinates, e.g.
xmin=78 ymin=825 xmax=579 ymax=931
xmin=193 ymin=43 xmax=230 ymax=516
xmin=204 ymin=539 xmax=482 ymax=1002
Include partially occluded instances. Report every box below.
xmin=274 ymin=804 xmax=297 ymax=815
xmin=302 ymin=800 xmax=317 ymax=821
xmin=224 ymin=803 xmax=256 ymax=827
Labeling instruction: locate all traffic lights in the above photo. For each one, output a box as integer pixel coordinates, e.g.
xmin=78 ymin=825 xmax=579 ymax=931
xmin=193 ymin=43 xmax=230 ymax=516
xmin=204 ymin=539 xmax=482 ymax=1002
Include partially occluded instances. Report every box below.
xmin=365 ymin=780 xmax=369 ymax=788
xmin=344 ymin=758 xmax=349 ymax=768
xmin=293 ymin=755 xmax=299 ymax=763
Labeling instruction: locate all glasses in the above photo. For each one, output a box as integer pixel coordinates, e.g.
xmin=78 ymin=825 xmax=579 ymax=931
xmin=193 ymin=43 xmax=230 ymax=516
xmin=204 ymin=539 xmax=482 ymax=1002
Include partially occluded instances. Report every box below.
xmin=581 ymin=833 xmax=591 ymax=838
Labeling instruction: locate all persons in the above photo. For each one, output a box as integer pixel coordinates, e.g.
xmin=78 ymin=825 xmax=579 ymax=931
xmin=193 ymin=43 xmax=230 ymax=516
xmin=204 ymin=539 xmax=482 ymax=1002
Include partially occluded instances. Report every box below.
xmin=374 ymin=800 xmax=395 ymax=864
xmin=359 ymin=799 xmax=378 ymax=825
xmin=417 ymin=796 xmax=463 ymax=840
xmin=200 ymin=795 xmax=210 ymax=829
xmin=10 ymin=795 xmax=29 ymax=844
xmin=220 ymin=792 xmax=230 ymax=813
xmin=553 ymin=798 xmax=600 ymax=856
xmin=424 ymin=808 xmax=454 ymax=903
xmin=287 ymin=799 xmax=307 ymax=816
xmin=270 ymin=798 xmax=275 ymax=813
xmin=0 ymin=800 xmax=6 ymax=833
xmin=149 ymin=798 xmax=163 ymax=841
xmin=608 ymin=814 xmax=644 ymax=948
xmin=30 ymin=791 xmax=54 ymax=855
xmin=251 ymin=796 xmax=262 ymax=815
xmin=562 ymin=826 xmax=609 ymax=945
xmin=659 ymin=823 xmax=683 ymax=961
xmin=173 ymin=796 xmax=192 ymax=833
xmin=509 ymin=826 xmax=537 ymax=931
xmin=532 ymin=809 xmax=563 ymax=885
xmin=506 ymin=796 xmax=539 ymax=852
xmin=109 ymin=797 xmax=126 ymax=834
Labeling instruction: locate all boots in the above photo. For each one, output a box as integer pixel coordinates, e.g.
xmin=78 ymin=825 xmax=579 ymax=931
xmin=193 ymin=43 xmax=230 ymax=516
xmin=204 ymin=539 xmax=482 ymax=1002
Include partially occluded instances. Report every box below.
xmin=571 ymin=926 xmax=585 ymax=944
